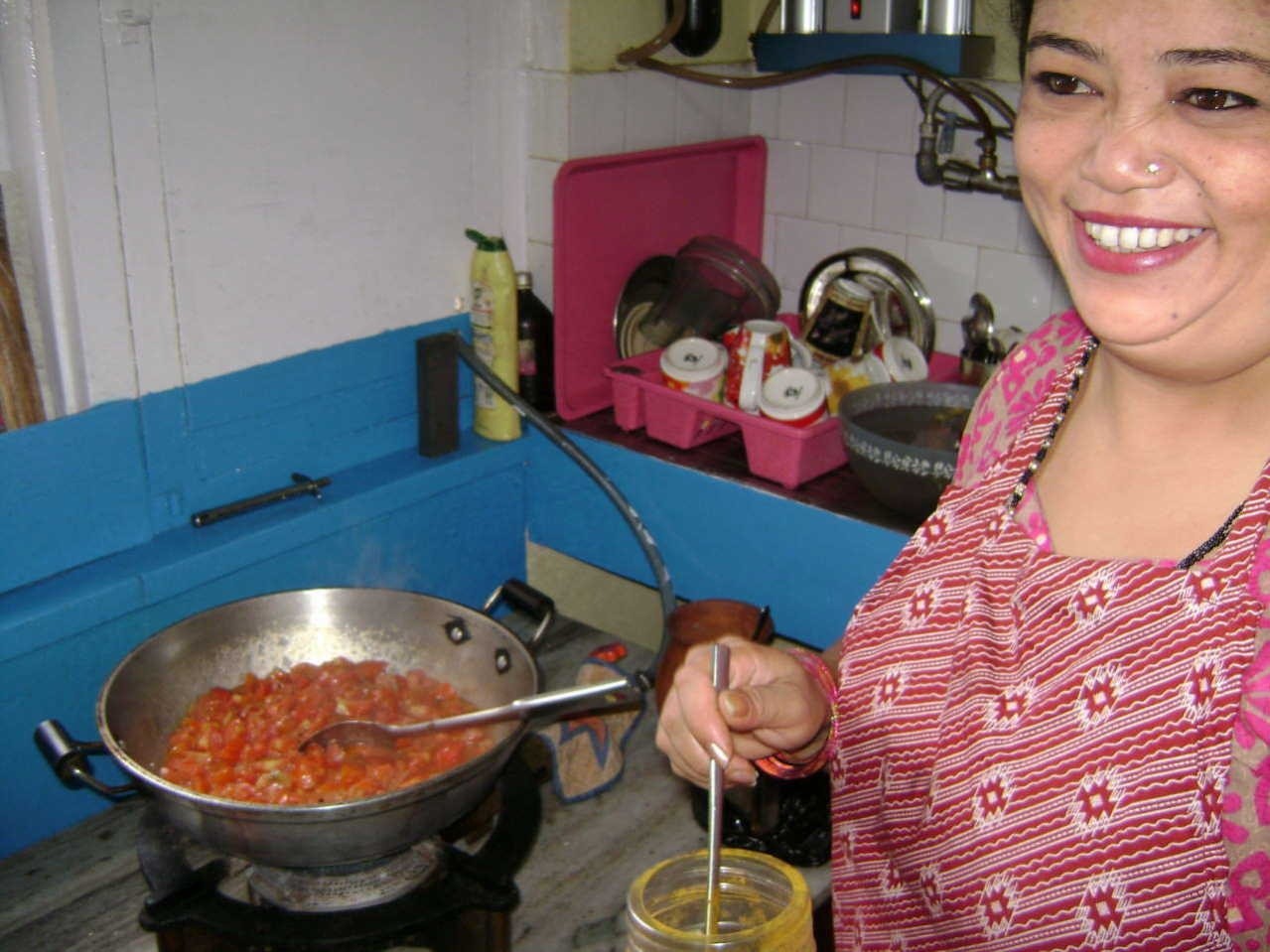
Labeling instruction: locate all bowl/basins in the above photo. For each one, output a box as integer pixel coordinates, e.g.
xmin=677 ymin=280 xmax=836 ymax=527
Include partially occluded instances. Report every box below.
xmin=837 ymin=381 xmax=981 ymax=521
xmin=611 ymin=255 xmax=676 ymax=360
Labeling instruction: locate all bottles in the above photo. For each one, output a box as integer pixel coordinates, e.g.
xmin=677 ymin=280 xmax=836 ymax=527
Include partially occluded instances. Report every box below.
xmin=623 ymin=847 xmax=817 ymax=952
xmin=464 ymin=226 xmax=521 ymax=442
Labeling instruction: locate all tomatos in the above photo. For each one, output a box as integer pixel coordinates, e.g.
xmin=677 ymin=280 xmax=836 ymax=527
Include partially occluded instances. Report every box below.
xmin=159 ymin=660 xmax=492 ymax=806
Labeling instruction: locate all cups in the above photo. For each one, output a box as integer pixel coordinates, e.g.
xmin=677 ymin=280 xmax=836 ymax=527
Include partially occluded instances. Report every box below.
xmin=871 ymin=336 xmax=929 ymax=383
xmin=801 ymin=277 xmax=875 ymax=366
xmin=755 ymin=366 xmax=829 ymax=431
xmin=657 ymin=597 xmax=775 ymax=714
xmin=721 ymin=317 xmax=794 ymax=417
xmin=659 ymin=337 xmax=729 ymax=405
xmin=635 ymin=232 xmax=782 ymax=343
xmin=818 ymin=351 xmax=892 ymax=418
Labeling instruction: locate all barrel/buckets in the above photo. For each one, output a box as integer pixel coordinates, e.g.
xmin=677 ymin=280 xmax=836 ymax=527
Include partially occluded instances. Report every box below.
xmin=515 ymin=271 xmax=554 ymax=414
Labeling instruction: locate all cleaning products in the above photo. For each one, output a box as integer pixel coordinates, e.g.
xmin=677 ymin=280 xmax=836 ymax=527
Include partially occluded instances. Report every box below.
xmin=462 ymin=227 xmax=525 ymax=442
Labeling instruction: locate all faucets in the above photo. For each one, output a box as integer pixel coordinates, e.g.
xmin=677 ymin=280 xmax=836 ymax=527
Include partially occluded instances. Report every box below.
xmin=960 ymin=293 xmax=1000 ymax=372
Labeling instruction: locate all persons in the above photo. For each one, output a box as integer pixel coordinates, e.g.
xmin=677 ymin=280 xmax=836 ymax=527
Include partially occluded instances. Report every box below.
xmin=657 ymin=0 xmax=1270 ymax=952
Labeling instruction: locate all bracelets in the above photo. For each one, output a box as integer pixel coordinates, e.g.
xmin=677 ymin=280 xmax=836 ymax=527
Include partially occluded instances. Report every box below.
xmin=753 ymin=646 xmax=842 ymax=779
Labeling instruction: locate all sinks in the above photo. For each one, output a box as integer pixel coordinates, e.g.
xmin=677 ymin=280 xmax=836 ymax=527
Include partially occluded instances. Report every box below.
xmin=834 ymin=379 xmax=981 ymax=516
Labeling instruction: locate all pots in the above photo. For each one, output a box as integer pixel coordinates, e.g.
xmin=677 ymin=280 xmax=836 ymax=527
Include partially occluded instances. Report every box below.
xmin=32 ymin=576 xmax=556 ymax=868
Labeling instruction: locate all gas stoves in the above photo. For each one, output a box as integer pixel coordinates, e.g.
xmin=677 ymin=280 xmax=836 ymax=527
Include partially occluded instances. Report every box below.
xmin=133 ymin=755 xmax=544 ymax=952
